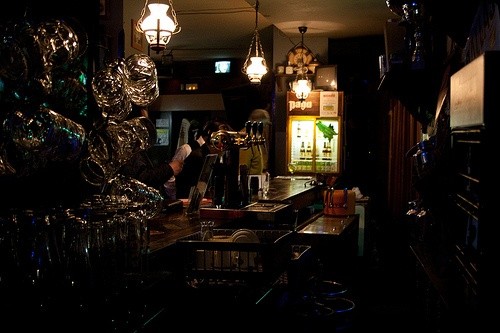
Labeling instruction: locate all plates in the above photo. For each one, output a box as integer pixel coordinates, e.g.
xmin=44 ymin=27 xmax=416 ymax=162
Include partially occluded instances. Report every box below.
xmin=229 ymin=228 xmax=259 ymax=269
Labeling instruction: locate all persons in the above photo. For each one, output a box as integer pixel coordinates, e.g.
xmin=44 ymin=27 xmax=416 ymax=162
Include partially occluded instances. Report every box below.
xmin=127 ymin=107 xmax=184 ymax=200
xmin=173 ymin=109 xmax=270 ymax=199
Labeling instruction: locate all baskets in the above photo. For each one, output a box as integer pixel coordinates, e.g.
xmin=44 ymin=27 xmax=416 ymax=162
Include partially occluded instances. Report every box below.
xmin=176 ymin=228 xmax=294 ymax=279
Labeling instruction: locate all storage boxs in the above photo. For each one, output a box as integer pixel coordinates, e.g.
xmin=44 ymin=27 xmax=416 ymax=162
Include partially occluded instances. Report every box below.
xmin=178 ymin=227 xmax=311 ymax=299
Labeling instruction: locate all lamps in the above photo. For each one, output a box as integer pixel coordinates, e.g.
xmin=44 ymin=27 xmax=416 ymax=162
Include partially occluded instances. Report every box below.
xmin=136 ymin=0 xmax=181 ymax=53
xmin=242 ymin=0 xmax=270 ymax=85
xmin=291 ymin=26 xmax=312 ymax=103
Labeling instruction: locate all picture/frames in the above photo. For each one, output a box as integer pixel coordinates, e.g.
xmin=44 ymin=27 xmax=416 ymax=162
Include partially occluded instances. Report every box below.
xmin=131 ymin=18 xmax=145 ymax=52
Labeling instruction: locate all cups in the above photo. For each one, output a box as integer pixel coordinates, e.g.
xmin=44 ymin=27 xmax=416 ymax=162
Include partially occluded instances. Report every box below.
xmin=1 ymin=20 xmax=163 ymax=226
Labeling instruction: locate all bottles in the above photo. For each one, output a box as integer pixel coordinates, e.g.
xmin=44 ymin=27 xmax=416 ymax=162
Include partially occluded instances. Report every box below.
xmin=306 ymin=141 xmax=312 ymax=159
xmin=300 ymin=142 xmax=306 ymax=160
xmin=322 ymin=142 xmax=332 ymax=160
